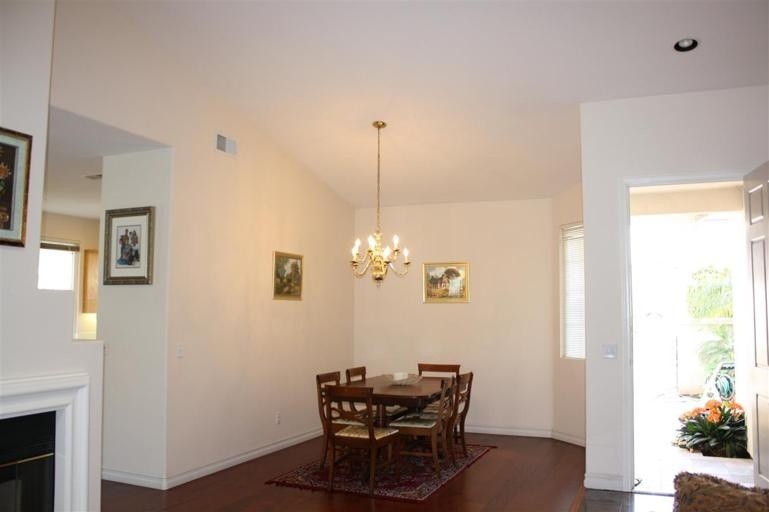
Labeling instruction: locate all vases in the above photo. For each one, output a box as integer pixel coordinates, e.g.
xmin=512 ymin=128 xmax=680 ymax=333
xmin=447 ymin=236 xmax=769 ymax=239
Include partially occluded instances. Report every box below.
xmin=669 ymin=398 xmax=749 ymax=457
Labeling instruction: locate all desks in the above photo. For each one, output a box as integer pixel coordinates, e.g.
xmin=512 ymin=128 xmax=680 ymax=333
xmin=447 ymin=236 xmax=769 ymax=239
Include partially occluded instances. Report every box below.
xmin=342 ymin=372 xmax=455 ymax=470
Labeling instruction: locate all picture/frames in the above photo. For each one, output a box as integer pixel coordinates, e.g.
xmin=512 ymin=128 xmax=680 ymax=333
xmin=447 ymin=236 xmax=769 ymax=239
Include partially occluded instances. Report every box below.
xmin=0 ymin=128 xmax=34 ymax=248
xmin=423 ymin=261 xmax=470 ymax=303
xmin=271 ymin=252 xmax=306 ymax=300
xmin=102 ymin=206 xmax=155 ymax=285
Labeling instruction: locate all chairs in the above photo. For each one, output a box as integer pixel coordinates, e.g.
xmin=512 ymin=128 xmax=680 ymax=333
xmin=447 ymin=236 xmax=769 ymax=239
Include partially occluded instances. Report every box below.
xmin=416 ymin=362 xmax=460 ymax=378
xmin=316 ymin=371 xmax=372 ymax=471
xmin=424 ymin=373 xmax=472 ymax=462
xmin=322 ymin=383 xmax=396 ymax=502
xmin=346 ymin=365 xmax=384 ymax=418
xmin=390 ymin=377 xmax=458 ymax=482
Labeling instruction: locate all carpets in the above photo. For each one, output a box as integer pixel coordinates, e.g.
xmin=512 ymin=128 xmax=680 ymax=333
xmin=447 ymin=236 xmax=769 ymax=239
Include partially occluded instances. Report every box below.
xmin=263 ymin=442 xmax=496 ymax=502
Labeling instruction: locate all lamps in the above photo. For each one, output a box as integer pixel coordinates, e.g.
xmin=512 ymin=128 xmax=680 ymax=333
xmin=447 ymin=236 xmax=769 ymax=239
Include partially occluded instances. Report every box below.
xmin=350 ymin=122 xmax=411 ymax=282
